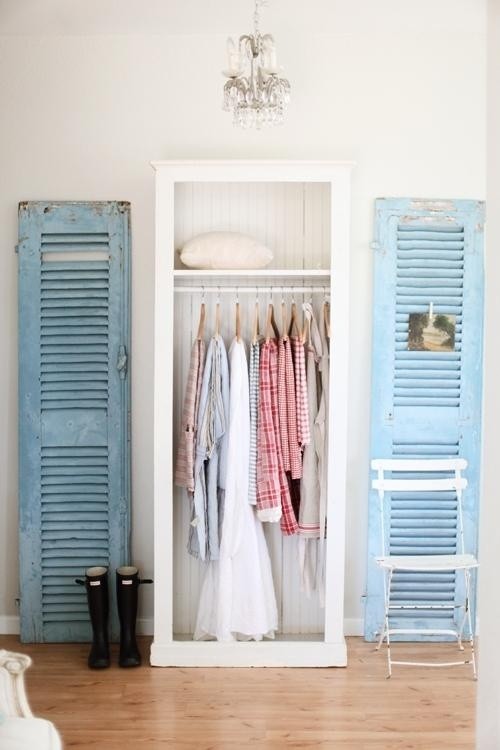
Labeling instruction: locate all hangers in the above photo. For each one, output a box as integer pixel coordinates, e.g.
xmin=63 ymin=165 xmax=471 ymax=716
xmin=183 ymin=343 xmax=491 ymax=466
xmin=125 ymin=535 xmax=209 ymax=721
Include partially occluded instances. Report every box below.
xmin=191 ymin=285 xmax=328 ymax=347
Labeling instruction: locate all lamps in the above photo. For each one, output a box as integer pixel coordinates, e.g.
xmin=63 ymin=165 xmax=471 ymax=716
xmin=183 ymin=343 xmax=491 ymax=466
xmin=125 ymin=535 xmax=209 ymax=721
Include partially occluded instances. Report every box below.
xmin=217 ymin=2 xmax=295 ymax=131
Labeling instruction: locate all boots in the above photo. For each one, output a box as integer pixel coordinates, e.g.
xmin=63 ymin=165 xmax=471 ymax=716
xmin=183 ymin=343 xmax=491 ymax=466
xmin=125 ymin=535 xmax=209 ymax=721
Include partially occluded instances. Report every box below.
xmin=74 ymin=566 xmax=110 ymax=670
xmin=114 ymin=564 xmax=153 ymax=670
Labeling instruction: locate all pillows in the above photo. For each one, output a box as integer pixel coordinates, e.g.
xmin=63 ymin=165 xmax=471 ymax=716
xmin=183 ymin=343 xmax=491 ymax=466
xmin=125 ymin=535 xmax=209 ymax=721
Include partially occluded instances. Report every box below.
xmin=179 ymin=226 xmax=275 ymax=275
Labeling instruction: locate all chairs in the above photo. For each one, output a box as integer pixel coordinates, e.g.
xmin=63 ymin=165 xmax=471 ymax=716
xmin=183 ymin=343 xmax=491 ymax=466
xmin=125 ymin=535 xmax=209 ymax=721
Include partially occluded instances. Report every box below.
xmin=371 ymin=455 xmax=477 ymax=675
xmin=1 ymin=649 xmax=63 ymax=750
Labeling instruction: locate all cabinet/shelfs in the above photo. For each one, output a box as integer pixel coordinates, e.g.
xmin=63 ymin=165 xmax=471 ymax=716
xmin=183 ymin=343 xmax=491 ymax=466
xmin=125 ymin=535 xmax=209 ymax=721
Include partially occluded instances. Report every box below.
xmin=145 ymin=158 xmax=352 ymax=670
